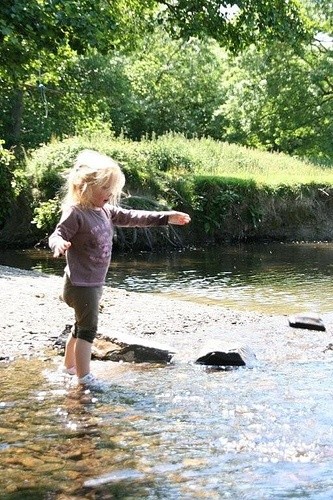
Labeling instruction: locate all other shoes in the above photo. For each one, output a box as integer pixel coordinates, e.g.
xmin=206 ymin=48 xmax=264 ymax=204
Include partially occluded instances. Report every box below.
xmin=62 ymin=365 xmax=78 ymax=374
xmin=80 ymin=375 xmax=106 ymax=388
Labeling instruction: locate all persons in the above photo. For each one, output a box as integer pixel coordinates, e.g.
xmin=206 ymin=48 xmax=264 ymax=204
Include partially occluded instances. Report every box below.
xmin=47 ymin=147 xmax=192 ymax=383
